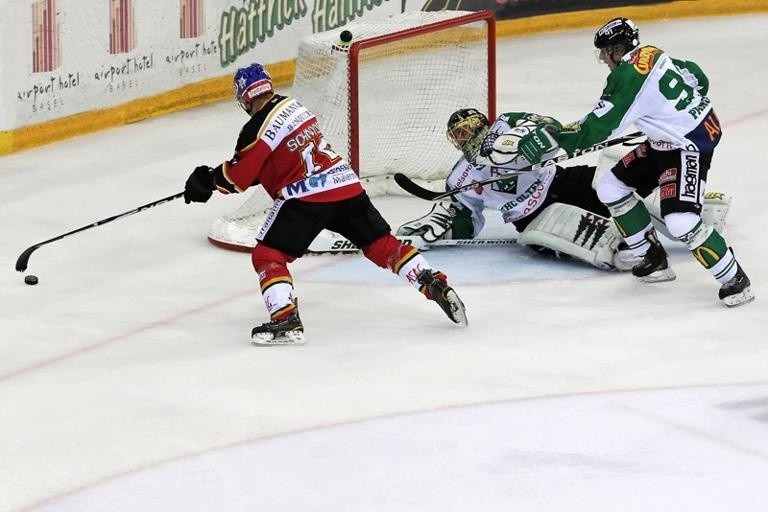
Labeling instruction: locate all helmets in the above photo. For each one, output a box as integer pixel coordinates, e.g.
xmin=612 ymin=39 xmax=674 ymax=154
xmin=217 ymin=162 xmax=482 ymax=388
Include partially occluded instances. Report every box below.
xmin=231 ymin=59 xmax=274 ymax=113
xmin=592 ymin=16 xmax=643 ymax=66
xmin=444 ymin=106 xmax=492 ymax=160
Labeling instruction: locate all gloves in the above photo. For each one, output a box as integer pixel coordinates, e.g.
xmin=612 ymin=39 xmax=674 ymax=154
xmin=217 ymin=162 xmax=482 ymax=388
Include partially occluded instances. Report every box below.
xmin=515 ymin=121 xmax=560 ymax=166
xmin=182 ymin=164 xmax=218 ymax=205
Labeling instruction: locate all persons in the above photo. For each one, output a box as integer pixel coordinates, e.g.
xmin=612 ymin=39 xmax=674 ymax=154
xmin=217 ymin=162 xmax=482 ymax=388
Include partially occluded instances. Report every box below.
xmin=183 ymin=63 xmax=466 ymax=339
xmin=394 ymin=107 xmax=729 ymax=272
xmin=527 ymin=16 xmax=751 ymax=299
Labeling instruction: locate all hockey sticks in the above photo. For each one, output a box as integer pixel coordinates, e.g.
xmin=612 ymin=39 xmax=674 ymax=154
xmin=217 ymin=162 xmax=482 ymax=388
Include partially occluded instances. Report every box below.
xmin=394 ymin=130 xmax=646 ymax=201
xmin=15 ymin=190 xmax=186 ymax=272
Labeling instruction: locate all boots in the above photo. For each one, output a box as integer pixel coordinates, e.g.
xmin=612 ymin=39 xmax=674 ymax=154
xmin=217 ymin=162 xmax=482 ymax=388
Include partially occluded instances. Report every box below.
xmin=631 ymin=232 xmax=670 ymax=276
xmin=715 ymin=245 xmax=752 ymax=299
xmin=248 ymin=313 xmax=306 ymax=340
xmin=416 ymin=269 xmax=466 ymax=325
